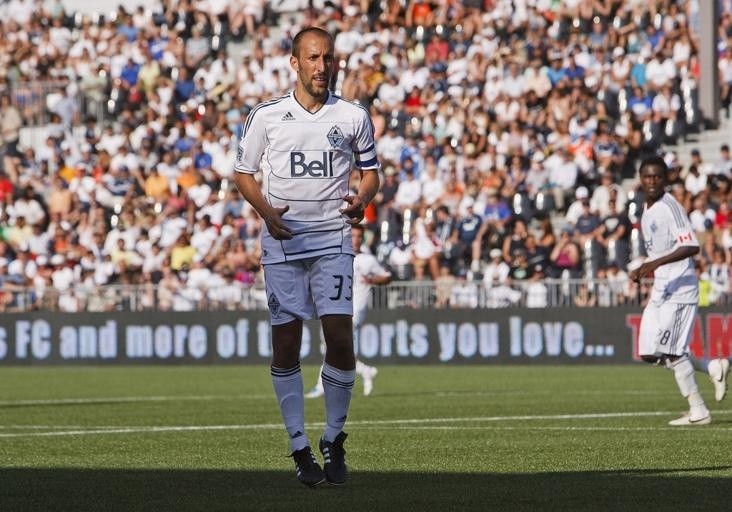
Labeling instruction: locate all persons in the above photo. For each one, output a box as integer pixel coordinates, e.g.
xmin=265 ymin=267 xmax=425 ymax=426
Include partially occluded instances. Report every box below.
xmin=1 ymin=0 xmax=732 ymax=314
xmin=234 ymin=26 xmax=381 ymax=486
xmin=630 ymin=157 xmax=730 ymax=426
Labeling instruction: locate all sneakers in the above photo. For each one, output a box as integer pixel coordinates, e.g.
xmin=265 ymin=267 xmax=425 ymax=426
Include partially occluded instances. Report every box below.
xmin=706 ymin=358 xmax=729 ymax=402
xmin=292 ymin=446 xmax=326 ymax=487
xmin=320 ymin=432 xmax=349 ymax=485
xmin=303 ymin=386 xmax=326 ymax=399
xmin=668 ymin=410 xmax=712 ymax=425
xmin=362 ymin=366 xmax=378 ymax=396
